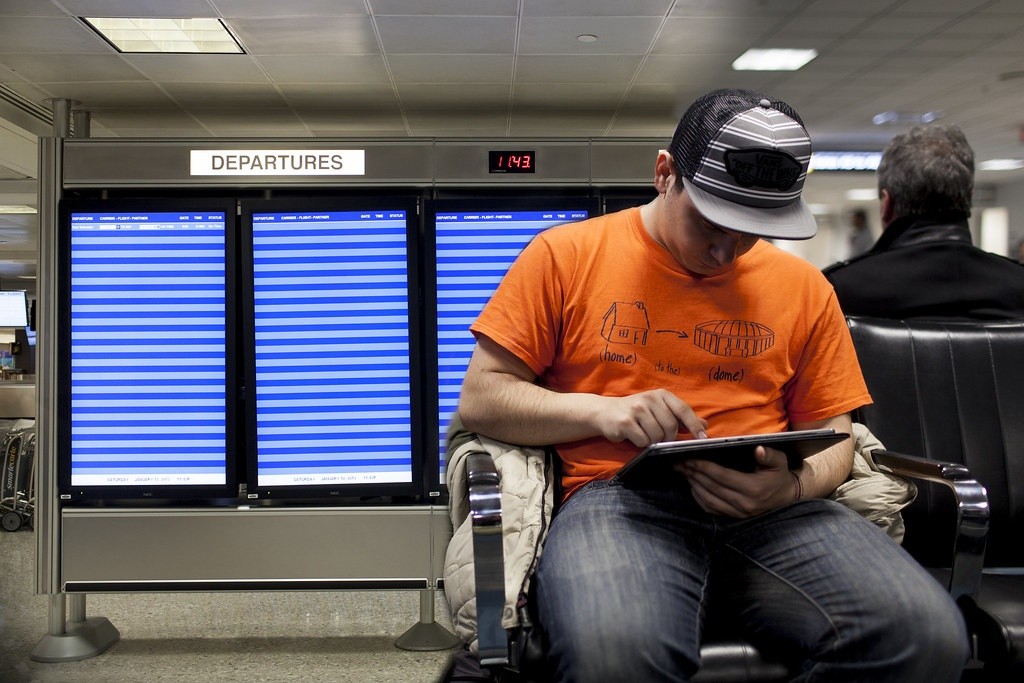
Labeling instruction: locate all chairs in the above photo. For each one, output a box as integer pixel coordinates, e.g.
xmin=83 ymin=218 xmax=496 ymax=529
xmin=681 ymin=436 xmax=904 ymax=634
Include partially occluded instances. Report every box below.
xmin=455 ymin=410 xmax=992 ymax=683
xmin=845 ymin=314 xmax=1024 ymax=569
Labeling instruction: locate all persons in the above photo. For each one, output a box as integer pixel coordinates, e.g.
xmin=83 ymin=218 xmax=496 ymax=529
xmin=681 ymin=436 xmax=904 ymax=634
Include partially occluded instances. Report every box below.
xmin=822 ymin=123 xmax=1024 ymax=319
xmin=459 ymin=90 xmax=968 ymax=683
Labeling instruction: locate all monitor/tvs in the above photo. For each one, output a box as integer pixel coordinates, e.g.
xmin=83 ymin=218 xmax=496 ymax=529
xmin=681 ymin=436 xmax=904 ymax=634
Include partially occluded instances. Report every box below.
xmin=0 ymin=290 xmax=29 ymax=327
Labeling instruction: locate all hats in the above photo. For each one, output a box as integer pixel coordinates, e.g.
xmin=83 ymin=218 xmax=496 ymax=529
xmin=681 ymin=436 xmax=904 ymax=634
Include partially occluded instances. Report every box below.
xmin=668 ymin=88 xmax=819 ymax=241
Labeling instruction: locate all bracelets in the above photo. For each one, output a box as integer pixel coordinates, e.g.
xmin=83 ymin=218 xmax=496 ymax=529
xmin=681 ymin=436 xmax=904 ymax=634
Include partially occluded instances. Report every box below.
xmin=791 ymin=472 xmax=803 ymax=503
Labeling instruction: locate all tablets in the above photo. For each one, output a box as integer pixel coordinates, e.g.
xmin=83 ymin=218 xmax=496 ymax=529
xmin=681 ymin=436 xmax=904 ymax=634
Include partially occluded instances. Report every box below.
xmin=608 ymin=428 xmax=850 ymax=487
